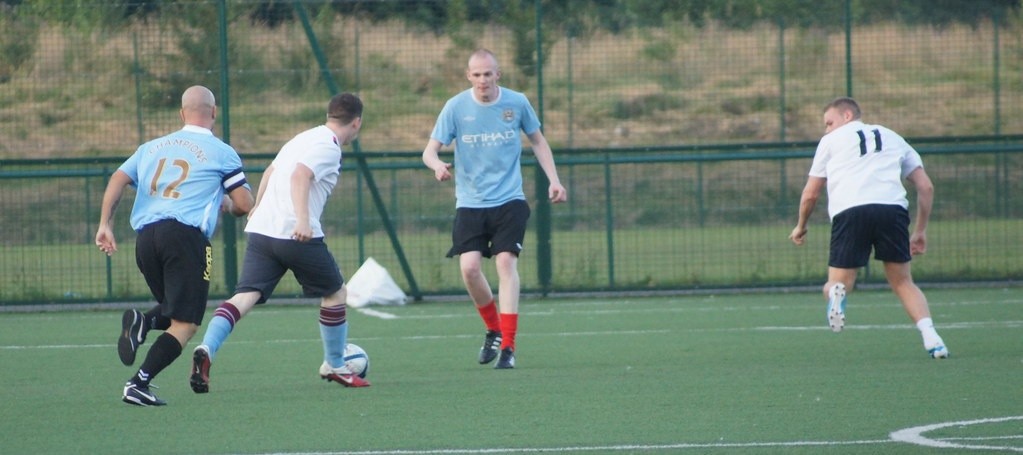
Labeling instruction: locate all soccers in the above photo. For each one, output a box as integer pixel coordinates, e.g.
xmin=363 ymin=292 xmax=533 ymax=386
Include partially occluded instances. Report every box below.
xmin=323 ymin=343 xmax=369 ymax=382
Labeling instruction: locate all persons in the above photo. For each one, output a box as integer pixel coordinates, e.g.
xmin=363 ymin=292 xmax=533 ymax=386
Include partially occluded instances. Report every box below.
xmin=94 ymin=86 xmax=254 ymax=408
xmin=190 ymin=93 xmax=370 ymax=394
xmin=788 ymin=98 xmax=949 ymax=358
xmin=422 ymin=48 xmax=568 ymax=370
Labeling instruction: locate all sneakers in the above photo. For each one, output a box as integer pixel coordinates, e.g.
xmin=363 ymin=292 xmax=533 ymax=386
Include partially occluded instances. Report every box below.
xmin=827 ymin=284 xmax=847 ymax=333
xmin=479 ymin=329 xmax=502 ymax=363
xmin=190 ymin=345 xmax=211 ymax=394
xmin=494 ymin=346 xmax=515 ymax=369
xmin=320 ymin=361 xmax=370 ymax=388
xmin=118 ymin=309 xmax=146 ymax=366
xmin=121 ymin=381 xmax=167 ymax=407
xmin=926 ymin=344 xmax=950 ymax=359
